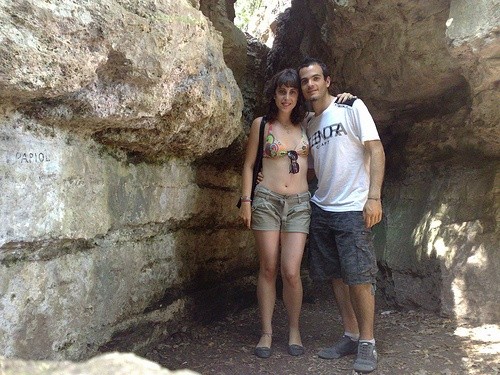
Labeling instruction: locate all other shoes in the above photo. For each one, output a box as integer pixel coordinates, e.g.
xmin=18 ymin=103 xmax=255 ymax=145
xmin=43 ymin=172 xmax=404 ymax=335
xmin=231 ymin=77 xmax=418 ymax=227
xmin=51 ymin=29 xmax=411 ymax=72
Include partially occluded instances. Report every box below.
xmin=255 ymin=346 xmax=271 ymax=358
xmin=288 ymin=342 xmax=304 ymax=356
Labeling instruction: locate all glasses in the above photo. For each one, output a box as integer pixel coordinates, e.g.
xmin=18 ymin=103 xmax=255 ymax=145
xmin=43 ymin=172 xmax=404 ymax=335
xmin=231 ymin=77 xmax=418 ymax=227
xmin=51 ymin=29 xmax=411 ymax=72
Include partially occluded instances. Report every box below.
xmin=287 ymin=150 xmax=300 ymax=174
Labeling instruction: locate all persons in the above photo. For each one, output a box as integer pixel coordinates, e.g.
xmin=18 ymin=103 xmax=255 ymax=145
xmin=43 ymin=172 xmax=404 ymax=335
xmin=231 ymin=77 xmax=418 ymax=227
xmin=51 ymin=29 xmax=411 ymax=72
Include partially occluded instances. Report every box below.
xmin=237 ymin=66 xmax=358 ymax=361
xmin=255 ymin=57 xmax=387 ymax=373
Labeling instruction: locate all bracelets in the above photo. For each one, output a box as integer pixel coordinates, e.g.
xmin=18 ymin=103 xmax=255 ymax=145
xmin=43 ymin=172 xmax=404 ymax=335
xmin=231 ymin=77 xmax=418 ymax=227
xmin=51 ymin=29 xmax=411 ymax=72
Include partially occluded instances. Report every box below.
xmin=239 ymin=197 xmax=253 ymax=202
xmin=367 ymin=196 xmax=382 ymax=201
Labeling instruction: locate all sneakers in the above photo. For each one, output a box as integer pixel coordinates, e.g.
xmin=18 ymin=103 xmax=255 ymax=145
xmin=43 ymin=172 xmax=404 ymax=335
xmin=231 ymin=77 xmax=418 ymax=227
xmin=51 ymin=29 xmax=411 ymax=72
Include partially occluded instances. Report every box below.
xmin=316 ymin=335 xmax=358 ymax=359
xmin=354 ymin=341 xmax=378 ymax=372
